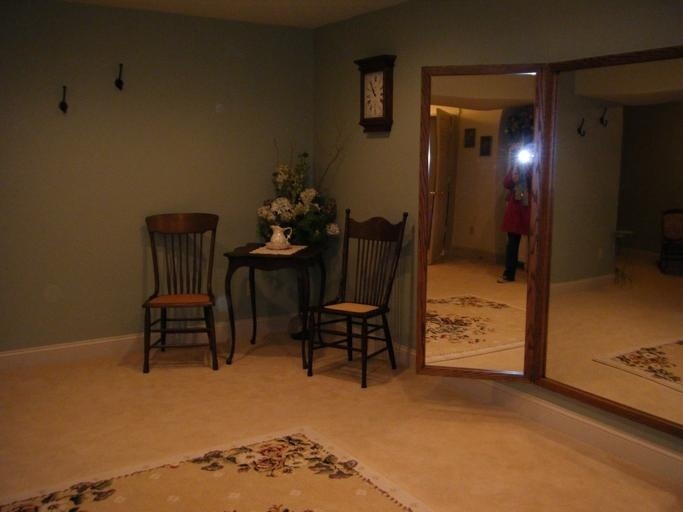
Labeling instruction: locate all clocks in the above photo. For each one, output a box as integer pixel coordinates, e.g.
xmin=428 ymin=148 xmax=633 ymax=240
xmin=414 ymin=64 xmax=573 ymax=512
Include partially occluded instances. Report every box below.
xmin=352 ymin=53 xmax=396 ymax=125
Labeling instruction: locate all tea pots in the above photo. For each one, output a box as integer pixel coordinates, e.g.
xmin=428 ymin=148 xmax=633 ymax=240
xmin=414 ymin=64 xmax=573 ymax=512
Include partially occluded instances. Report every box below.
xmin=268 ymin=224 xmax=293 ymax=250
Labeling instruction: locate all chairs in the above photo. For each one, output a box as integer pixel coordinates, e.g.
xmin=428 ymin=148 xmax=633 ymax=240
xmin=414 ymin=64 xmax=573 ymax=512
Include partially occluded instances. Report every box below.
xmin=655 ymin=208 xmax=682 ymax=274
xmin=140 ymin=213 xmax=219 ymax=374
xmin=305 ymin=208 xmax=408 ymax=388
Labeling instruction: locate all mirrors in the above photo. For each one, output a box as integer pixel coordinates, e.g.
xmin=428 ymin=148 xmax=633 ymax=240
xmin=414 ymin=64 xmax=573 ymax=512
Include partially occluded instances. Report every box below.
xmin=416 ymin=45 xmax=682 ymax=442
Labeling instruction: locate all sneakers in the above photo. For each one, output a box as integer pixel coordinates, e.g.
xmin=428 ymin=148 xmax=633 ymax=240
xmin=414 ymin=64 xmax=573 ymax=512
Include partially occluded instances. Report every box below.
xmin=497 ymin=276 xmax=508 ymax=283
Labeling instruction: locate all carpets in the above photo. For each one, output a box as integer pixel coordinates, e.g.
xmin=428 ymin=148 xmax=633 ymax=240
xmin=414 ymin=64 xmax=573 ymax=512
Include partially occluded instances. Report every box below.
xmin=593 ymin=326 xmax=682 ymax=393
xmin=425 ymin=294 xmax=525 ymax=363
xmin=0 ymin=427 xmax=429 ymax=512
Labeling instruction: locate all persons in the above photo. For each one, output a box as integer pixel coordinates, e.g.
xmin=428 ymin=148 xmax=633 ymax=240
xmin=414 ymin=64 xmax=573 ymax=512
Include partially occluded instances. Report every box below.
xmin=495 ymin=145 xmax=533 ymax=284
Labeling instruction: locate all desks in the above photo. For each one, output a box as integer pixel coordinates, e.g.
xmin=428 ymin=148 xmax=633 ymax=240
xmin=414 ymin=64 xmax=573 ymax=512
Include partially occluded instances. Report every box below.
xmin=222 ymin=242 xmax=310 ymax=370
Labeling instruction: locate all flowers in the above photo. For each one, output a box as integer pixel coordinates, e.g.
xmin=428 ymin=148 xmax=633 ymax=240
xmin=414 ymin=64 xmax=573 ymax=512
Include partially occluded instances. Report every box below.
xmin=252 ymin=118 xmax=349 ymax=246
